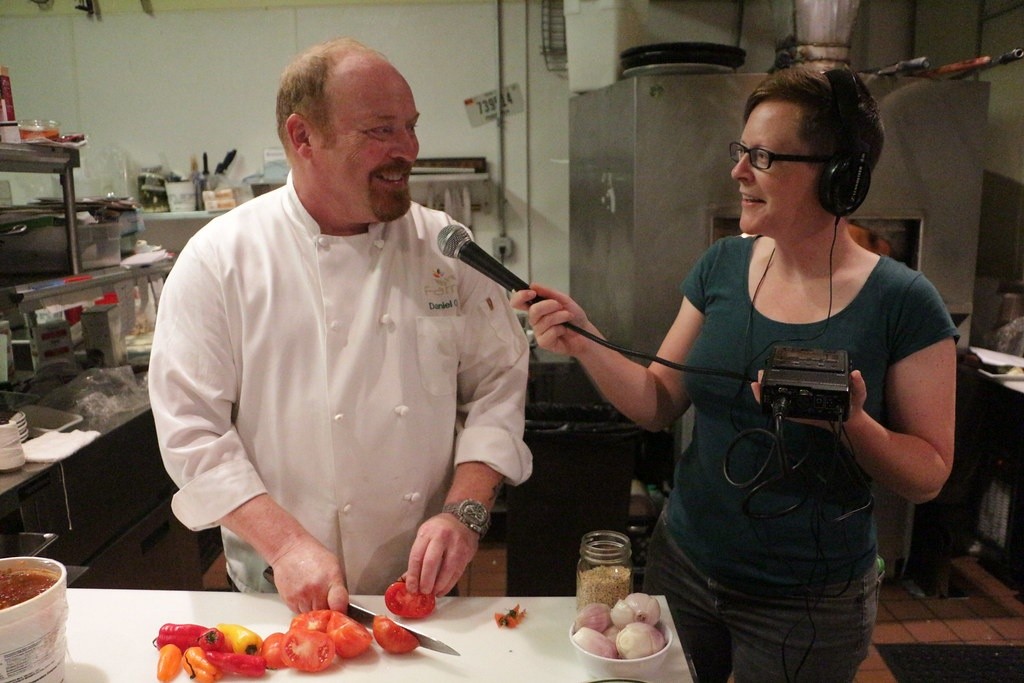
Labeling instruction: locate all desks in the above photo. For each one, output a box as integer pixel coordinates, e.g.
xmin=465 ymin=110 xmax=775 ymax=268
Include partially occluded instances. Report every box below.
xmin=63 ymin=589 xmax=695 ymax=683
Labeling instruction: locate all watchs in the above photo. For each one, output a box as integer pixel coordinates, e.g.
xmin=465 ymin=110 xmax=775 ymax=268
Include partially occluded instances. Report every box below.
xmin=441 ymin=498 xmax=492 ymax=543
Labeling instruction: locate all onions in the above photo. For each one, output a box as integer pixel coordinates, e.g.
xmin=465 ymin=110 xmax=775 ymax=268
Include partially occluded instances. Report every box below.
xmin=572 ymin=593 xmax=666 ymax=660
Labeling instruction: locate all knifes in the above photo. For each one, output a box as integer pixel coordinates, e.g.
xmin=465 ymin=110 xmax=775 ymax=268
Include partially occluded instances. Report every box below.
xmin=263 ymin=564 xmax=462 ymax=661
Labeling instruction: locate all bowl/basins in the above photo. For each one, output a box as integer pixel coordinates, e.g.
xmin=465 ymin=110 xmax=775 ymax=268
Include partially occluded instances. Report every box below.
xmin=20 ymin=119 xmax=61 ymax=143
xmin=570 ymin=617 xmax=673 ymax=676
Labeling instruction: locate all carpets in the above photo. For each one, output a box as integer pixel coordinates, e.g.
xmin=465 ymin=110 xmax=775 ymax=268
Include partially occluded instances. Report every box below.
xmin=874 ymin=642 xmax=1024 ymax=683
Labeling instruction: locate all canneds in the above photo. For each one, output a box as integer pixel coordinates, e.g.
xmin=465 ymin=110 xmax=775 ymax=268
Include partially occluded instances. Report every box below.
xmin=575 ymin=530 xmax=633 ymax=613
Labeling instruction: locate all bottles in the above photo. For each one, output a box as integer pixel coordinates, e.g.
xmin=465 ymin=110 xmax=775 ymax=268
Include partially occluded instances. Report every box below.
xmin=138 ymin=166 xmax=168 ymax=213
xmin=577 ymin=531 xmax=633 ymax=613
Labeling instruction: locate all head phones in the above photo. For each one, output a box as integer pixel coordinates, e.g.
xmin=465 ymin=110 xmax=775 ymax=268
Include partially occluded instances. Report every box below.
xmin=817 ymin=67 xmax=871 ymax=218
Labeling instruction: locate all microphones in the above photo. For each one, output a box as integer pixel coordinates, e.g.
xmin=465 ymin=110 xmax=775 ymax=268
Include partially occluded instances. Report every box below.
xmin=437 ymin=225 xmax=545 ymax=307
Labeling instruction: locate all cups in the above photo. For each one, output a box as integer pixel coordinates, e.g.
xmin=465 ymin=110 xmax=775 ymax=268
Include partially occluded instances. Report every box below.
xmin=165 ymin=182 xmax=198 ymax=211
xmin=0 ymin=556 xmax=67 ymax=683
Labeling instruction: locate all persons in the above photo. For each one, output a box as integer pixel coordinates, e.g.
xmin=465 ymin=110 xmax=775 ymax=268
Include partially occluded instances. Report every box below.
xmin=147 ymin=39 xmax=536 ymax=620
xmin=509 ymin=62 xmax=961 ymax=679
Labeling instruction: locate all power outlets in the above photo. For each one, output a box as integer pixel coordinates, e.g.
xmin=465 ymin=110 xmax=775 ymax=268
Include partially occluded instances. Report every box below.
xmin=492 ymin=238 xmax=512 ymax=258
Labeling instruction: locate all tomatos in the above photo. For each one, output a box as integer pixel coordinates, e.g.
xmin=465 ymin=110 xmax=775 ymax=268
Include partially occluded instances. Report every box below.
xmin=374 ymin=615 xmax=420 ymax=654
xmin=258 ymin=611 xmax=373 ymax=671
xmin=385 ymin=576 xmax=436 ymax=618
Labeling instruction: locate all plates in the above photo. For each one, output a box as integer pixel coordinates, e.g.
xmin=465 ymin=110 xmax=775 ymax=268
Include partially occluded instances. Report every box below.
xmin=11 ymin=411 xmax=29 ymax=442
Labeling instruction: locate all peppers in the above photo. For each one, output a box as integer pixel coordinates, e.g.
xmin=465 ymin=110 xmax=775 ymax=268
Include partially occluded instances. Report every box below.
xmin=150 ymin=623 xmax=267 ymax=683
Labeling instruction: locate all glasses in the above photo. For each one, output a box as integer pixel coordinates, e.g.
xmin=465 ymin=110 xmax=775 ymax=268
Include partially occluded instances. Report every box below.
xmin=730 ymin=141 xmax=819 ymax=169
